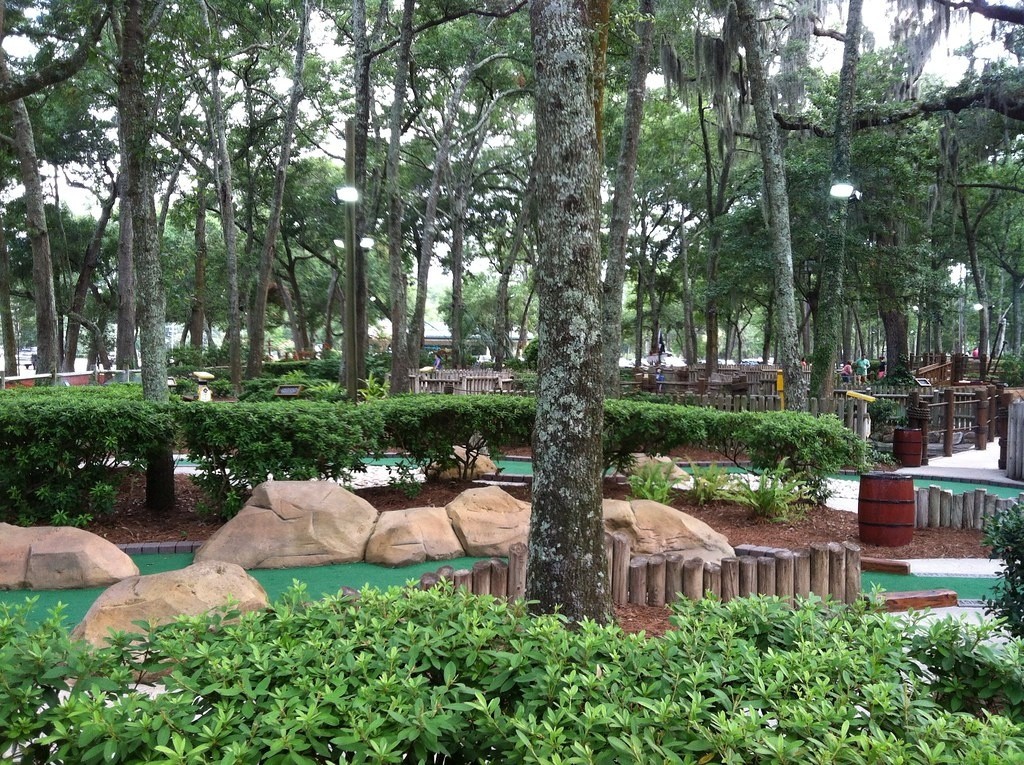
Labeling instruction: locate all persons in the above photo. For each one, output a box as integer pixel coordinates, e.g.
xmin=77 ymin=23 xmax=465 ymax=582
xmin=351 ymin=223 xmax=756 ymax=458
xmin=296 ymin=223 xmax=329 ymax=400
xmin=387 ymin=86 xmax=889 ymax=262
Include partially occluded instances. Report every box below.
xmin=801 ymin=357 xmax=806 ymax=366
xmin=855 ymin=354 xmax=870 ymax=386
xmin=841 ymin=360 xmax=853 ymax=383
xmin=877 ymin=357 xmax=887 ymax=380
xmin=972 ymin=347 xmax=978 ymax=357
xmin=433 ymin=353 xmax=442 ymax=372
xmin=655 ymin=368 xmax=665 ymax=393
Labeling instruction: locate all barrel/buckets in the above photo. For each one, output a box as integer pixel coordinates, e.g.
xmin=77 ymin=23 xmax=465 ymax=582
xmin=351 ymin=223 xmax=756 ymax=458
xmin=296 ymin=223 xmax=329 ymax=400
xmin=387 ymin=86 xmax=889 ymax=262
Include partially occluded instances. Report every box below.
xmin=858 ymin=474 xmax=914 ymax=547
xmin=893 ymin=429 xmax=923 ymax=467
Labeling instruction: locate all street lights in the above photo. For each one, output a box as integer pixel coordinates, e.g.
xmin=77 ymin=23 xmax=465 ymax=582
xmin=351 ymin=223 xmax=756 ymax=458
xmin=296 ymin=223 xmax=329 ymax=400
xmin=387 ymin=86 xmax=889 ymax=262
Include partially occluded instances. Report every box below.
xmin=808 ymin=180 xmax=863 ymax=414
xmin=329 ymin=186 xmax=374 ymax=405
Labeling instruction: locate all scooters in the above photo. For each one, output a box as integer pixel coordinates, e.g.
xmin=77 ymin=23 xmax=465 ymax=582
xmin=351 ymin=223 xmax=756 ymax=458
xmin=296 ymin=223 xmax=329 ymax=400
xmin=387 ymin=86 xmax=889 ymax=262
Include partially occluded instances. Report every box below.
xmin=168 ymin=371 xmax=218 ymax=402
